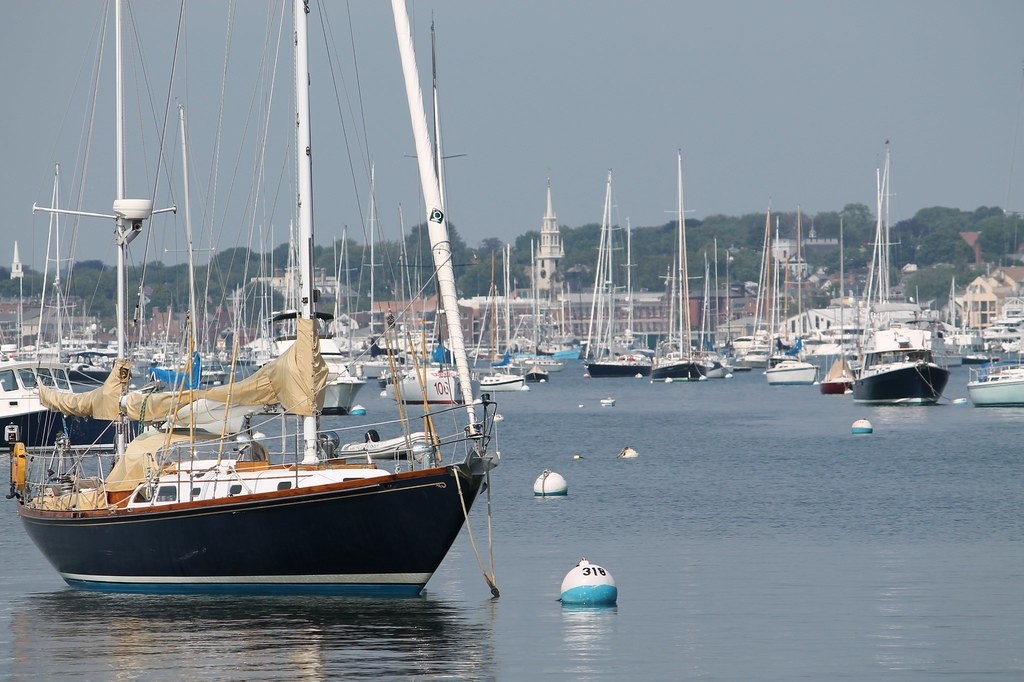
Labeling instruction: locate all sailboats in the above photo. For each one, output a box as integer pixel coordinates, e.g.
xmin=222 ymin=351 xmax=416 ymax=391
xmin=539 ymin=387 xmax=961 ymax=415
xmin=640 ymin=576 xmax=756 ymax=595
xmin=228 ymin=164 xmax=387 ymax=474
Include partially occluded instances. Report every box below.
xmin=5 ymin=1 xmax=499 ymax=600
xmin=820 ymin=218 xmax=857 ymax=394
xmin=256 ymin=219 xmax=367 ymax=415
xmin=766 ymin=204 xmax=821 ymax=385
xmin=651 ymin=148 xmax=733 ymax=383
xmin=345 ymin=162 xmax=439 ymax=379
xmin=479 ymin=244 xmax=525 ymax=392
xmin=582 ymin=168 xmax=653 ymax=378
xmin=0 ymin=162 xmax=228 ymax=453
xmin=537 ymin=281 xmax=594 ymax=357
xmin=490 ymin=238 xmax=563 ymax=375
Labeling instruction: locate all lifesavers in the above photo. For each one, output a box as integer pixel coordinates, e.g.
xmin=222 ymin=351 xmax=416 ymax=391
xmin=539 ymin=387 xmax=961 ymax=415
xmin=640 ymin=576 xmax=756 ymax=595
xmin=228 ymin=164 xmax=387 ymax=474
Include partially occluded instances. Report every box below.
xmin=11 ymin=442 xmax=28 ymax=492
xmin=616 ymin=355 xmax=628 ymax=363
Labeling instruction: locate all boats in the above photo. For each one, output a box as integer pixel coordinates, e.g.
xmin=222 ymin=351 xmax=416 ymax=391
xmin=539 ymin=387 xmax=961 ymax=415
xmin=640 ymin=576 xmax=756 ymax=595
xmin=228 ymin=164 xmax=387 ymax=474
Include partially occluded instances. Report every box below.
xmin=851 ymin=329 xmax=951 ymax=406
xmin=966 ymin=365 xmax=1024 ymax=407
xmin=377 ymin=370 xmax=409 ymax=389
xmin=524 ymin=365 xmax=549 ymax=383
xmin=945 ymin=318 xmax=1024 ymax=352
xmin=479 ymin=345 xmax=584 ymax=360
xmin=386 ymin=367 xmax=480 ymax=404
